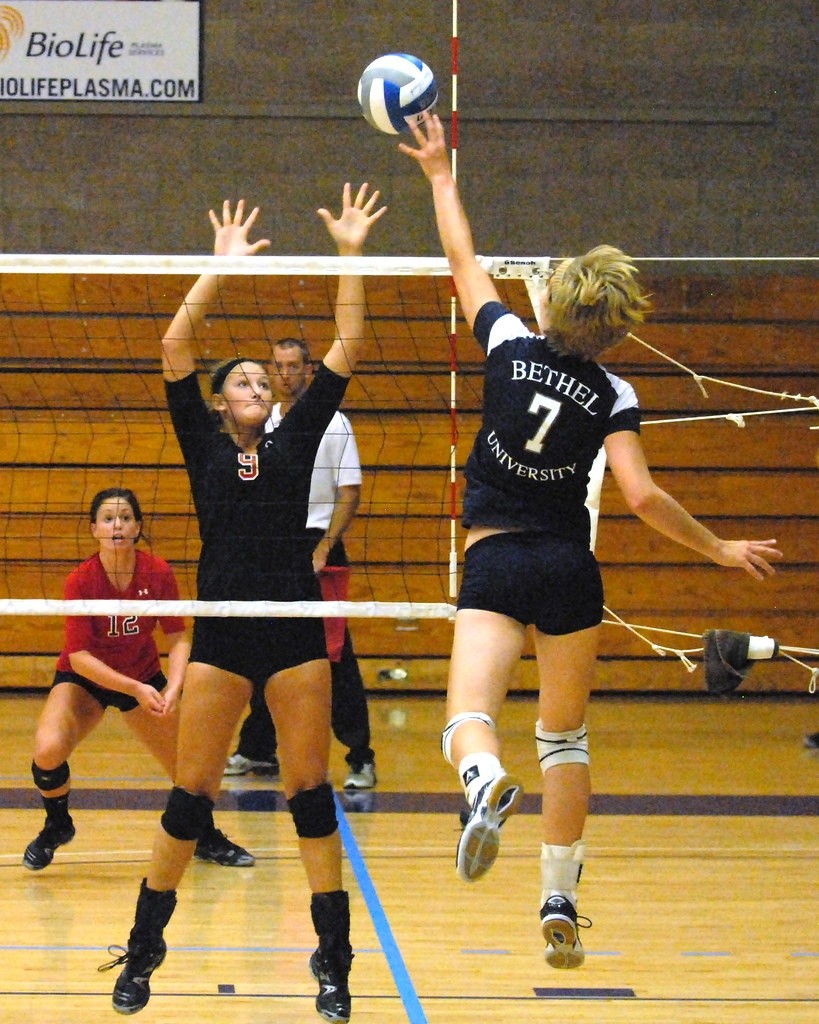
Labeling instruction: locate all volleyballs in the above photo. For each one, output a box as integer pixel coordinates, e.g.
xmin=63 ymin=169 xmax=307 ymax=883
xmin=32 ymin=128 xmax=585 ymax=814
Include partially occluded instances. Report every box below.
xmin=357 ymin=52 xmax=439 ymax=136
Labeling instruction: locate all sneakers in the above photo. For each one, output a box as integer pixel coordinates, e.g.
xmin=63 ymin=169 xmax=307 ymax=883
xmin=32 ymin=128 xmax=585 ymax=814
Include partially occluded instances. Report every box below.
xmin=97 ymin=937 xmax=166 ymax=1015
xmin=195 ymin=829 xmax=254 ymax=867
xmin=21 ymin=815 xmax=76 ymax=869
xmin=540 ymin=895 xmax=592 ymax=969
xmin=343 ymin=763 xmax=378 ymax=788
xmin=308 ymin=945 xmax=354 ymax=1023
xmin=456 ymin=774 xmax=524 ymax=883
xmin=224 ymin=754 xmax=279 ymax=776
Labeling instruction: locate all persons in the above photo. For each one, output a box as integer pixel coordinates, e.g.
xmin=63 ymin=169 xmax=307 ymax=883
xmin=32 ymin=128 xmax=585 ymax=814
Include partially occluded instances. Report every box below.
xmin=399 ymin=109 xmax=782 ymax=967
xmin=23 ymin=488 xmax=255 ymax=871
xmin=224 ymin=338 xmax=377 ymax=790
xmin=98 ymin=181 xmax=387 ymax=1024
